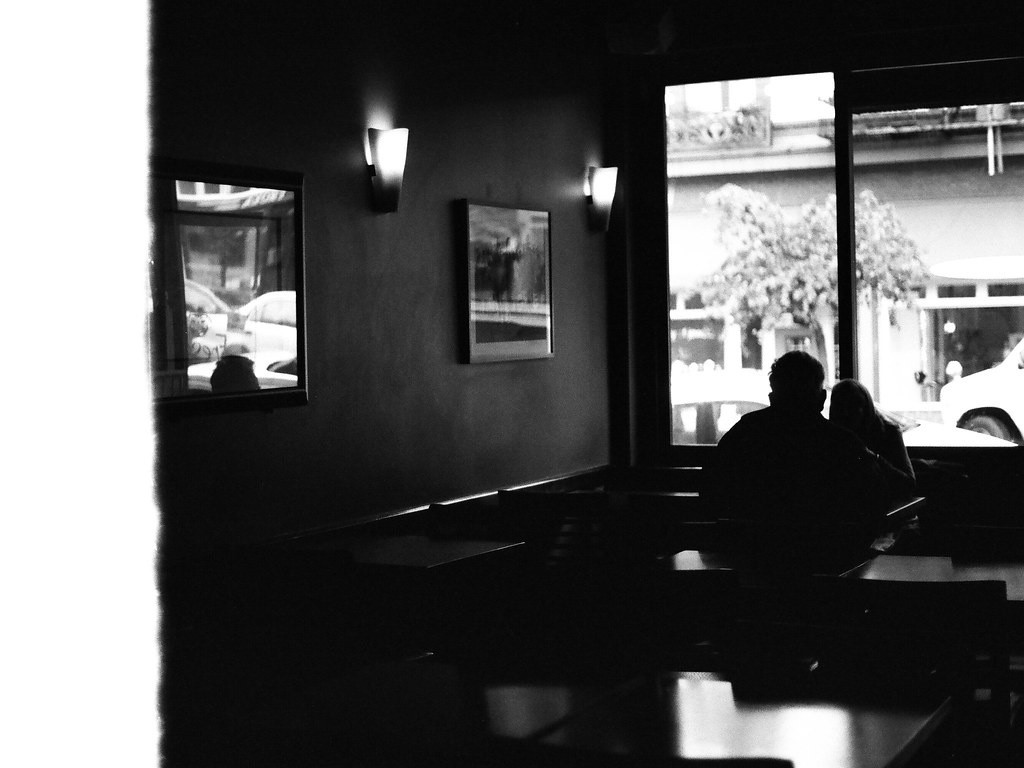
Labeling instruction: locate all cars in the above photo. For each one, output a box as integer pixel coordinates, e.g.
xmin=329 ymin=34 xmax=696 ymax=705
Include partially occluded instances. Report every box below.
xmin=234 ymin=291 xmax=296 ymax=327
xmin=186 ymin=348 xmax=297 ymax=389
xmin=472 ymin=299 xmax=550 ymax=345
xmin=185 ymin=279 xmax=297 ymax=348
xmin=672 ymin=367 xmax=1020 ymax=447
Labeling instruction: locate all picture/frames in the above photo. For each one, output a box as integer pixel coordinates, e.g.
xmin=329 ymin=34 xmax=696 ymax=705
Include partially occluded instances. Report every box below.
xmin=463 ymin=197 xmax=555 ymax=363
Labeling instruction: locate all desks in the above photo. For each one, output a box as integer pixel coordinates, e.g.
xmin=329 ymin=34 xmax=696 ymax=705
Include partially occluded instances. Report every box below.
xmin=163 ymin=456 xmax=1023 ymax=767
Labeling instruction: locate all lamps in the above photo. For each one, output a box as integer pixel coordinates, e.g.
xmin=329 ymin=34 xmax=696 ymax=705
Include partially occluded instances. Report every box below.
xmin=362 ymin=108 xmax=410 ymax=215
xmin=585 ymin=165 xmax=618 ymax=232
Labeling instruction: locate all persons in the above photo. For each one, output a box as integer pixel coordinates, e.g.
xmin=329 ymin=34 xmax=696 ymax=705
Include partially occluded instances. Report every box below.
xmin=699 ymin=351 xmax=922 ymax=555
xmin=210 ymin=355 xmax=262 ymax=392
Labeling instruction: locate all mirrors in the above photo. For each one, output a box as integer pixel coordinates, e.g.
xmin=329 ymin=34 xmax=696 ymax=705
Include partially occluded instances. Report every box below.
xmin=151 ymin=154 xmax=310 ymax=411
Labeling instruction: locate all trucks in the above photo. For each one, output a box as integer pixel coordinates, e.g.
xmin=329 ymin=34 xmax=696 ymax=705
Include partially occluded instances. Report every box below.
xmin=939 ymin=337 xmax=1023 ymax=444
